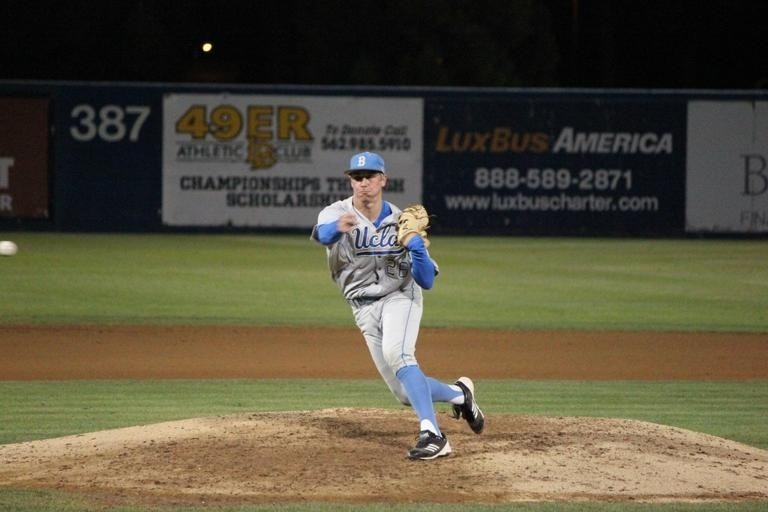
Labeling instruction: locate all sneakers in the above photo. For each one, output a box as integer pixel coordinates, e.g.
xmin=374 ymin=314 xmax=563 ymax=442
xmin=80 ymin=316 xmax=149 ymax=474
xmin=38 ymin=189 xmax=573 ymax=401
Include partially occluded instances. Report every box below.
xmin=449 ymin=376 xmax=485 ymax=435
xmin=407 ymin=429 xmax=452 ymax=461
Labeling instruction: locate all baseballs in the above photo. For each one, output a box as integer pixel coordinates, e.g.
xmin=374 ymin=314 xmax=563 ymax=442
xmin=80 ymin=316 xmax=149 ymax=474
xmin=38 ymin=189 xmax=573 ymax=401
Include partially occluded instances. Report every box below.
xmin=0 ymin=242 xmax=18 ymax=257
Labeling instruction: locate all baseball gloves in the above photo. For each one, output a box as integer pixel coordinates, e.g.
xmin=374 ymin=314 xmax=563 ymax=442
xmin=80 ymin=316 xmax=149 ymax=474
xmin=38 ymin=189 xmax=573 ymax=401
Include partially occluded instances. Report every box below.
xmin=397 ymin=203 xmax=429 ymax=245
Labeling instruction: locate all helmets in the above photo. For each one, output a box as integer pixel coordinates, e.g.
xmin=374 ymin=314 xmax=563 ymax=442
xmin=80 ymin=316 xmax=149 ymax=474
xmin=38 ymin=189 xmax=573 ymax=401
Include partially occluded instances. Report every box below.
xmin=343 ymin=152 xmax=385 ymax=178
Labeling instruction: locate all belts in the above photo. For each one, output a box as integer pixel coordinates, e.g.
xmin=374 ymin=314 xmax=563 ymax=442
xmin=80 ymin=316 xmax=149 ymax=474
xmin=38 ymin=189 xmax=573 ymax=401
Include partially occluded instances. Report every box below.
xmin=349 ymin=297 xmax=381 ymax=306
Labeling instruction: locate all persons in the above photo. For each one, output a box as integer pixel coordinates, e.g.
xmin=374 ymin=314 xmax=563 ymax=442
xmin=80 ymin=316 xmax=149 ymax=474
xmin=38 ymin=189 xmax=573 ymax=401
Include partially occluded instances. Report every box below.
xmin=308 ymin=151 xmax=486 ymax=461
